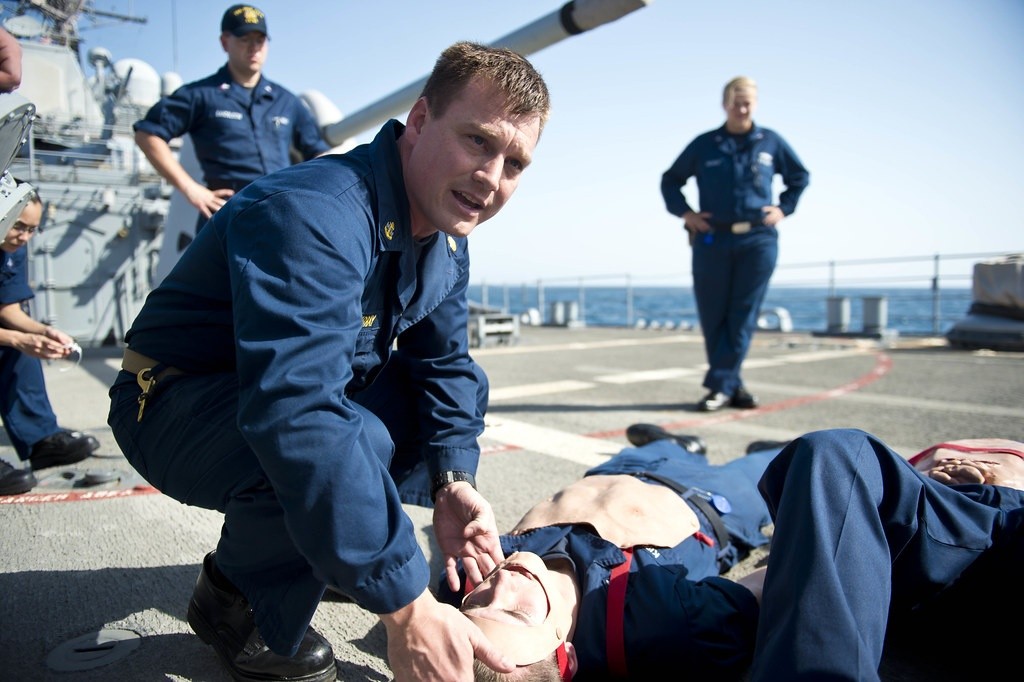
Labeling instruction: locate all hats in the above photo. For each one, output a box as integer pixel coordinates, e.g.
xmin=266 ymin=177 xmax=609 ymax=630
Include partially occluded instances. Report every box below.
xmin=222 ymin=5 xmax=270 ymax=42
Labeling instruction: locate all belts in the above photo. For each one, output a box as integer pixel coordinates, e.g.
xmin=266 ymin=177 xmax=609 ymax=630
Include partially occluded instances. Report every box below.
xmin=121 ymin=348 xmax=180 ymax=382
xmin=709 ymin=221 xmax=763 ymax=234
xmin=598 ymin=467 xmax=728 ymax=550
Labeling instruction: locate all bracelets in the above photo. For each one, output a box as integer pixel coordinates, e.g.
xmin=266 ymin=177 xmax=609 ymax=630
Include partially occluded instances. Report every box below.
xmin=430 ymin=471 xmax=476 ymax=504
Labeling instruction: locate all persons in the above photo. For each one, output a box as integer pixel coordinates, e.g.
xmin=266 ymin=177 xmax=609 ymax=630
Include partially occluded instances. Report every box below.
xmin=133 ymin=5 xmax=333 ymax=234
xmin=106 ymin=43 xmax=550 ymax=682
xmin=662 ymin=78 xmax=808 ymax=409
xmin=439 ymin=422 xmax=1024 ymax=682
xmin=0 ymin=30 xmax=99 ymax=497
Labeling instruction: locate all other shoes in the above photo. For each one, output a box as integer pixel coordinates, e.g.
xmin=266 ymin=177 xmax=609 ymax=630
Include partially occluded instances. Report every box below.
xmin=731 ymin=385 xmax=758 ymax=409
xmin=701 ymin=390 xmax=728 ymax=411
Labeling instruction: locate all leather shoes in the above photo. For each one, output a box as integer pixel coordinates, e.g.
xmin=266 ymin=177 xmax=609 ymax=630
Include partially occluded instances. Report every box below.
xmin=627 ymin=423 xmax=706 ymax=457
xmin=187 ymin=552 xmax=335 ymax=681
xmin=0 ymin=458 xmax=38 ymax=495
xmin=30 ymin=432 xmax=99 ymax=470
xmin=747 ymin=440 xmax=790 ymax=456
